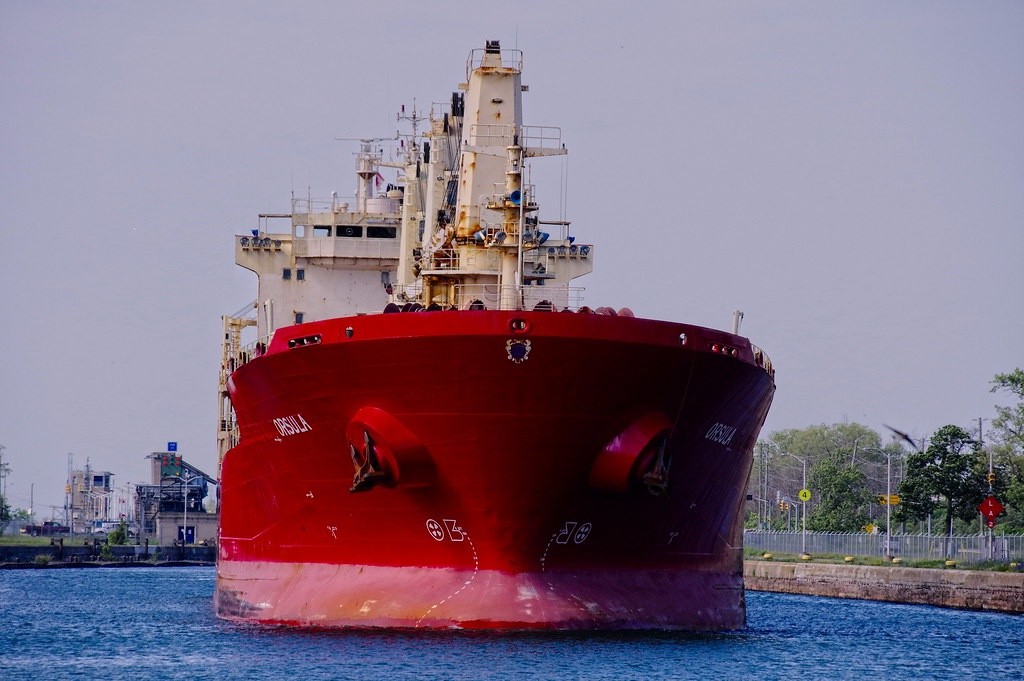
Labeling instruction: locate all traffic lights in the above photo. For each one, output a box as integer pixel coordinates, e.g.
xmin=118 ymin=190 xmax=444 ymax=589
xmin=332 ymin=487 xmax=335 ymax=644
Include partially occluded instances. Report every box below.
xmin=781 ymin=500 xmax=789 ymax=511
xmin=746 ymin=495 xmax=752 ymax=500
xmin=989 ymin=473 xmax=996 ymax=485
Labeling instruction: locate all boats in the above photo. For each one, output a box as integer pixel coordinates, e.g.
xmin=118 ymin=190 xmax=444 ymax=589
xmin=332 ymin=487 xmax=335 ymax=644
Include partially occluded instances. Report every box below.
xmin=212 ymin=39 xmax=775 ymax=635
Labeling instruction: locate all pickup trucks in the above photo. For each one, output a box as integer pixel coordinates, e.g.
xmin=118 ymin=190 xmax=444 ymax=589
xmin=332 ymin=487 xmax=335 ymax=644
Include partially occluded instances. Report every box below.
xmin=20 ymin=521 xmax=71 ymax=537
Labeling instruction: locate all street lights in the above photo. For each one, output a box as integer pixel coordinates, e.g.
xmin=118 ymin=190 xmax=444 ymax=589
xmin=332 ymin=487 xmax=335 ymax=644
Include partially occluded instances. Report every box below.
xmin=862 ymin=448 xmax=891 ymax=555
xmin=172 ymin=476 xmax=203 ymax=544
xmin=780 ymin=454 xmax=807 ymax=554
